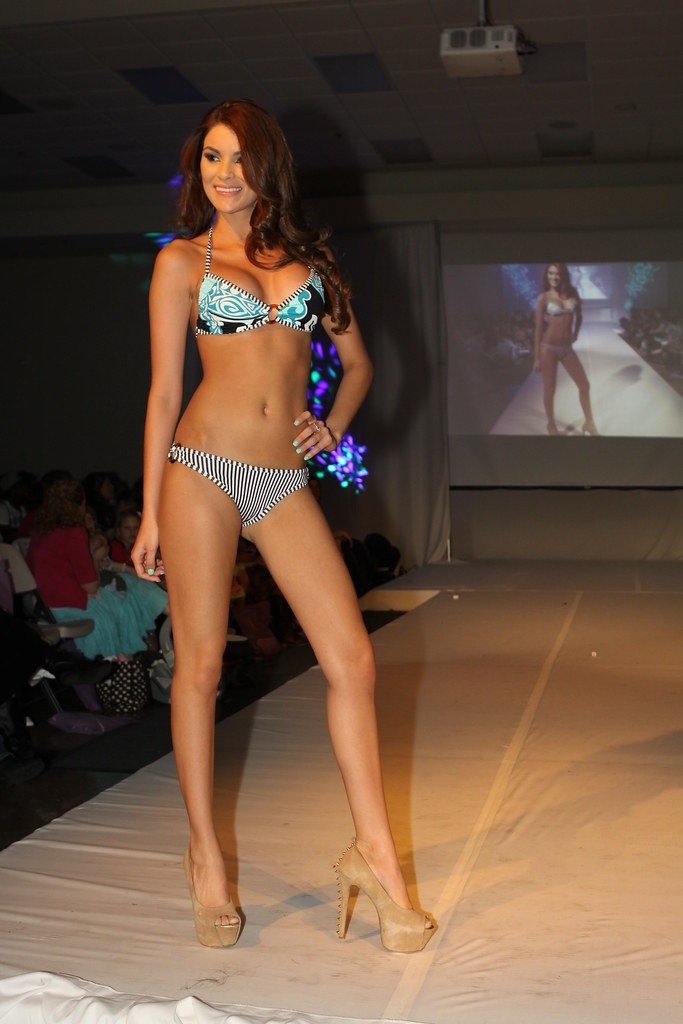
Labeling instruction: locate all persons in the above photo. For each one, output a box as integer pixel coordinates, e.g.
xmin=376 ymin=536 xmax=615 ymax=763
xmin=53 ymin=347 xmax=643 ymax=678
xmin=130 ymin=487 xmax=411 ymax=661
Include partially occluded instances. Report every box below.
xmin=468 ymin=308 xmax=533 ymax=369
xmin=0 ymin=466 xmax=404 ymax=785
xmin=617 ymin=304 xmax=679 ymax=359
xmin=129 ymin=98 xmax=439 ymax=957
xmin=532 ymin=264 xmax=603 ymax=436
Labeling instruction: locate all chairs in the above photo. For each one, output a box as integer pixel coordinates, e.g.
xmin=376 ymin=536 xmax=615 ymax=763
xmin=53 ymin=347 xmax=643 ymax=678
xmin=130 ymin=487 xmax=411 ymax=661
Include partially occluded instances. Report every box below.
xmin=1 ymin=543 xmax=95 ymax=714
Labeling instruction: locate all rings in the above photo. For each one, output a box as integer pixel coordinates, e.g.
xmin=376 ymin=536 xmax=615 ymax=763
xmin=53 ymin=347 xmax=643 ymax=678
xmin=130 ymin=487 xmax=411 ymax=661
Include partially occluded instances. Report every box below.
xmin=312 ymin=422 xmax=322 ymax=433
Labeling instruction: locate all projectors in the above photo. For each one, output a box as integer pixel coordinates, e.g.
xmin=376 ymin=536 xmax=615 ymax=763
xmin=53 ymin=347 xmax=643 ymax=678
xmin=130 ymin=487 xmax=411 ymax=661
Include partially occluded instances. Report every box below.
xmin=439 ymin=25 xmax=526 ymax=79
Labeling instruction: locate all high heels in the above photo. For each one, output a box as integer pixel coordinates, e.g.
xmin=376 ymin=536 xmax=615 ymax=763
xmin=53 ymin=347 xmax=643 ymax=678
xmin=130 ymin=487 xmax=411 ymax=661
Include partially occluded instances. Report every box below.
xmin=335 ymin=843 xmax=437 ymax=952
xmin=582 ymin=424 xmax=600 ymax=435
xmin=182 ymin=849 xmax=241 ymax=946
xmin=547 ymin=424 xmax=560 ymax=435
